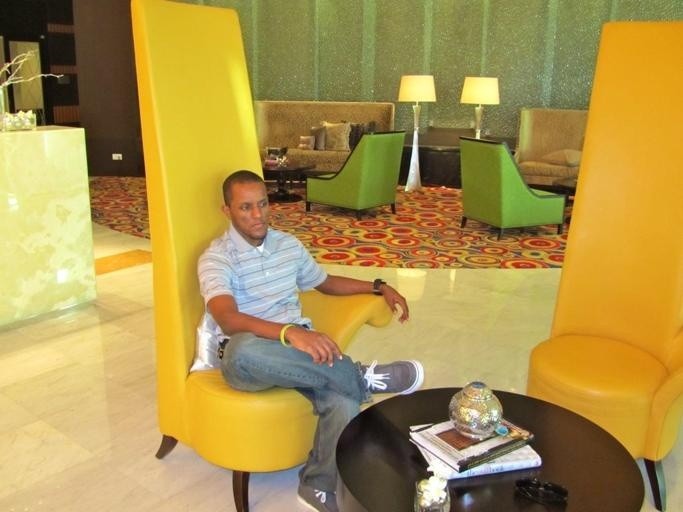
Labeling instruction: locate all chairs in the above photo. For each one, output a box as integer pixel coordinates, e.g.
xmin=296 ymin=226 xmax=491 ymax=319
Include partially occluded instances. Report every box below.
xmin=131 ymin=0 xmax=394 ymax=511
xmin=306 ymin=130 xmax=406 ymax=219
xmin=459 ymin=135 xmax=565 ymax=241
xmin=523 ymin=21 xmax=683 ymax=512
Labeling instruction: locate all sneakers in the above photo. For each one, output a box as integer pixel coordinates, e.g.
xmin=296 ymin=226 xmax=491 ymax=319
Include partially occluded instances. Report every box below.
xmin=296 ymin=482 xmax=339 ymax=512
xmin=361 ymin=360 xmax=424 ymax=395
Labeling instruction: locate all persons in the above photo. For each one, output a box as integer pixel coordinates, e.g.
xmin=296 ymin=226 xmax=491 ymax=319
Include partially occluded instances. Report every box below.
xmin=196 ymin=170 xmax=426 ymax=511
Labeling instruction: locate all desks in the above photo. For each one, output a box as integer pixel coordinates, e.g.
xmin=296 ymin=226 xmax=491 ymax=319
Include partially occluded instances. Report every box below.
xmin=334 ymin=384 xmax=645 ymax=512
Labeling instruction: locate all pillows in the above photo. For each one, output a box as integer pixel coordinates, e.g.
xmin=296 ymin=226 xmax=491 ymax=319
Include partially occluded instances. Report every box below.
xmin=543 ymin=148 xmax=582 ymax=167
xmin=297 ymin=120 xmax=377 ymax=154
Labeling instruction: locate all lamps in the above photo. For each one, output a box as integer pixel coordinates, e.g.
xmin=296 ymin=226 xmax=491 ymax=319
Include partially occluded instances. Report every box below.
xmin=396 ymin=75 xmax=436 ymax=191
xmin=458 ymin=76 xmax=499 ymax=141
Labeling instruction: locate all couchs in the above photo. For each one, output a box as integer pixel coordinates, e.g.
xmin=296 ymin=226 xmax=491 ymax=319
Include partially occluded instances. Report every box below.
xmin=252 ymin=100 xmax=396 ymax=175
xmin=512 ymin=107 xmax=588 ymax=192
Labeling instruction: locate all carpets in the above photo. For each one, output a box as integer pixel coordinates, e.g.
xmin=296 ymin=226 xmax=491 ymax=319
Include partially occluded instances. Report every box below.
xmin=88 ymin=174 xmax=577 ymax=268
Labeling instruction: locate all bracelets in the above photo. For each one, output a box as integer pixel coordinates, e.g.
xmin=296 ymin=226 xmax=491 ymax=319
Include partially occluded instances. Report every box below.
xmin=373 ymin=279 xmax=386 ymax=296
xmin=280 ymin=324 xmax=296 ymax=348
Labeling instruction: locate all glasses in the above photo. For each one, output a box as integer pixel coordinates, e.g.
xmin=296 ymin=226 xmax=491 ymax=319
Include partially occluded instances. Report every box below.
xmin=514 ymin=476 xmax=568 ymax=506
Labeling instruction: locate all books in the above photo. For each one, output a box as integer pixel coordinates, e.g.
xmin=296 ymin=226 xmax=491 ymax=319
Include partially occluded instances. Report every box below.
xmin=407 ymin=423 xmax=542 ymax=479
xmin=409 ymin=417 xmax=535 ymax=473
